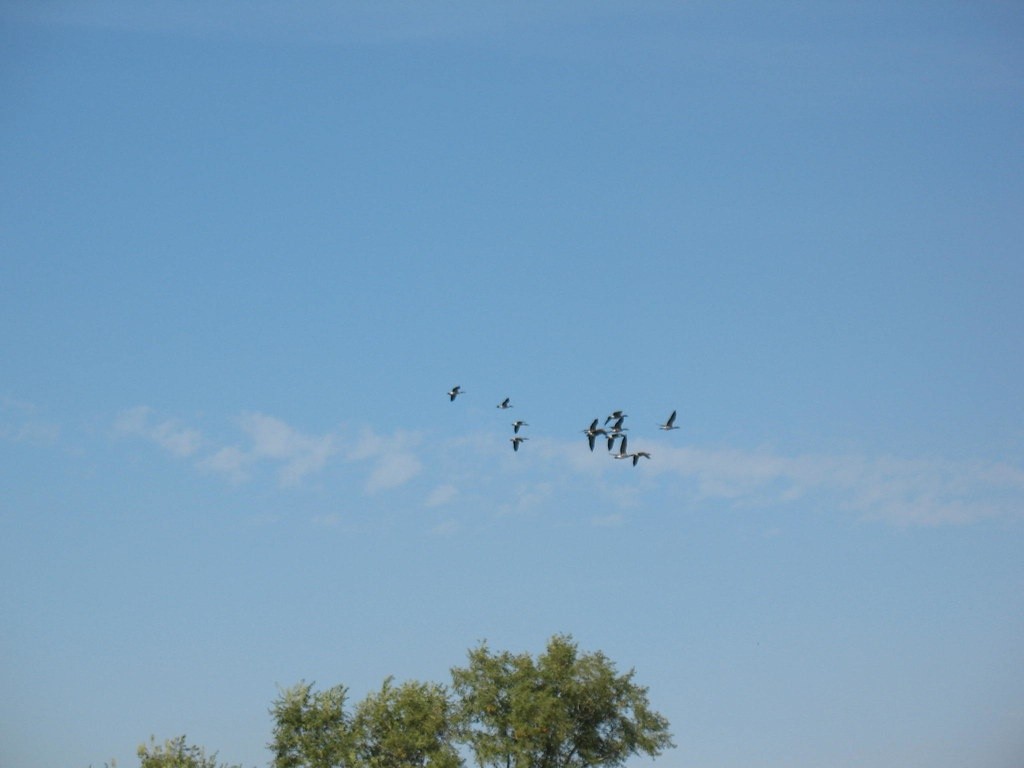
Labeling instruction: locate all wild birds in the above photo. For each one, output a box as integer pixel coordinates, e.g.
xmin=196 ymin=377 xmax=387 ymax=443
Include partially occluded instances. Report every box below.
xmin=447 ymin=386 xmax=682 ymax=467
